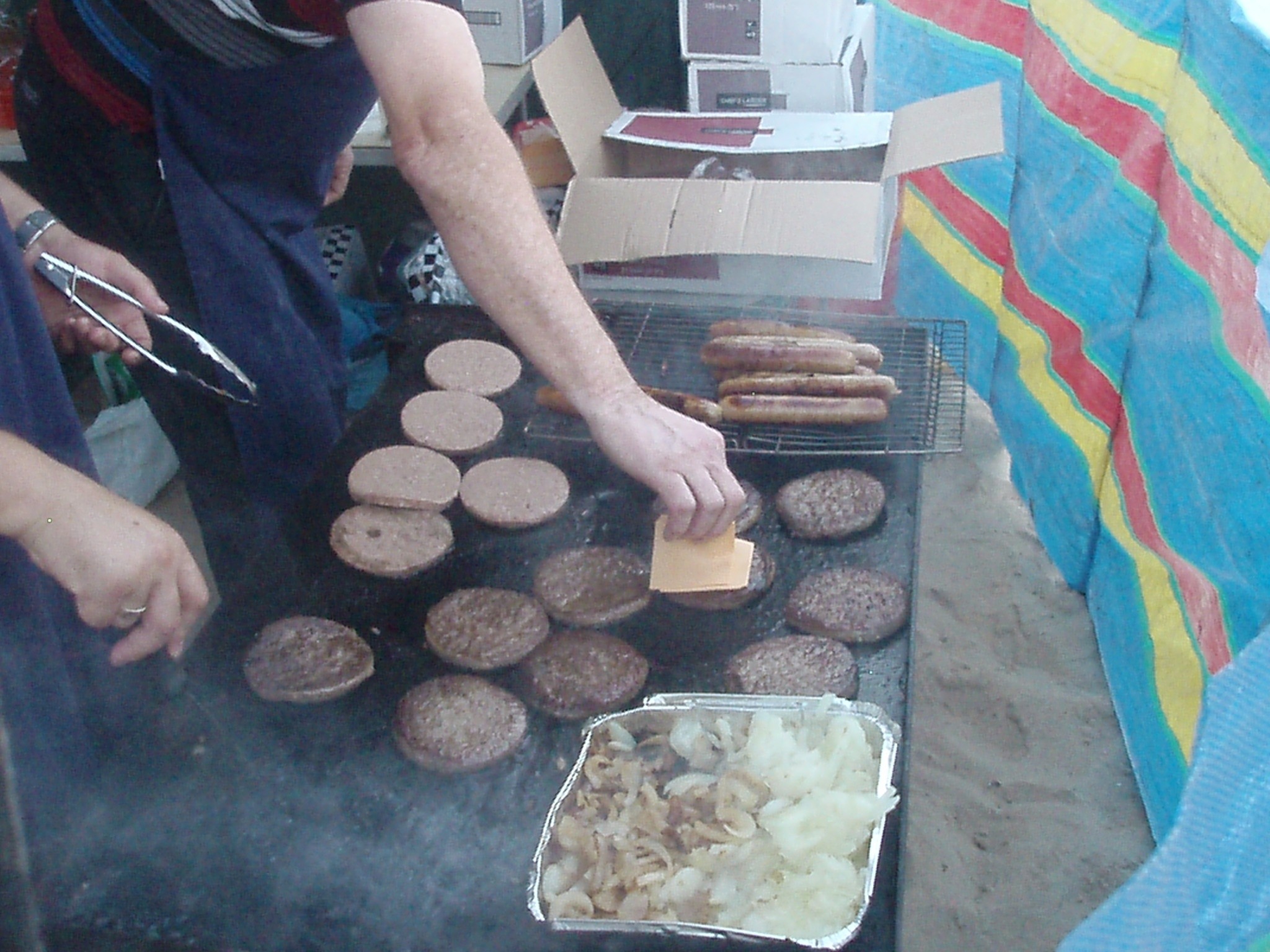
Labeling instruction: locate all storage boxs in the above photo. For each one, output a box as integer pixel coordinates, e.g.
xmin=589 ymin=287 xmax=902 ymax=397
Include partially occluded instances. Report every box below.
xmin=533 ymin=0 xmax=1006 ymax=319
xmin=462 ymin=0 xmax=562 ymax=67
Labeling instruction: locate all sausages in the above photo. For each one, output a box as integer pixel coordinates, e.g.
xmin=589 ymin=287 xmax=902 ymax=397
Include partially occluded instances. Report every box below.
xmin=532 ymin=384 xmax=723 ymax=425
xmin=701 ymin=318 xmax=898 ymax=425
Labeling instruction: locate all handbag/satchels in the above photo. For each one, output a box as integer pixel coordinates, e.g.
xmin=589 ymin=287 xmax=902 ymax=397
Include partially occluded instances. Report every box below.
xmin=84 ymin=352 xmax=221 ymax=654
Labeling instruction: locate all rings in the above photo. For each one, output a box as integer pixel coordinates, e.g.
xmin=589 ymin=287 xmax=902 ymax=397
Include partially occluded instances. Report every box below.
xmin=120 ymin=605 xmax=146 ymax=617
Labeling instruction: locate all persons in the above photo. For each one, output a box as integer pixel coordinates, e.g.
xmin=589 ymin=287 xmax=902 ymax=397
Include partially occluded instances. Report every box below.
xmin=0 ymin=168 xmax=211 ymax=890
xmin=12 ymin=0 xmax=747 ymax=602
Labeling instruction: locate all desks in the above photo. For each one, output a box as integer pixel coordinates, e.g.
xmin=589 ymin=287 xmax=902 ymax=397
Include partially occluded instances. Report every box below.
xmin=351 ymin=63 xmax=532 ymax=170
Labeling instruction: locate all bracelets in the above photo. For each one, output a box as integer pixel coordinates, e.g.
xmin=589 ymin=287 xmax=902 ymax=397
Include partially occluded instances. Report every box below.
xmin=14 ymin=210 xmax=64 ymax=254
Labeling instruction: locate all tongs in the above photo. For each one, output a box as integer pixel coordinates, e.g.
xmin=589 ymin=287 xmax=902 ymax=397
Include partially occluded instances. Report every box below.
xmin=33 ymin=251 xmax=259 ymax=406
